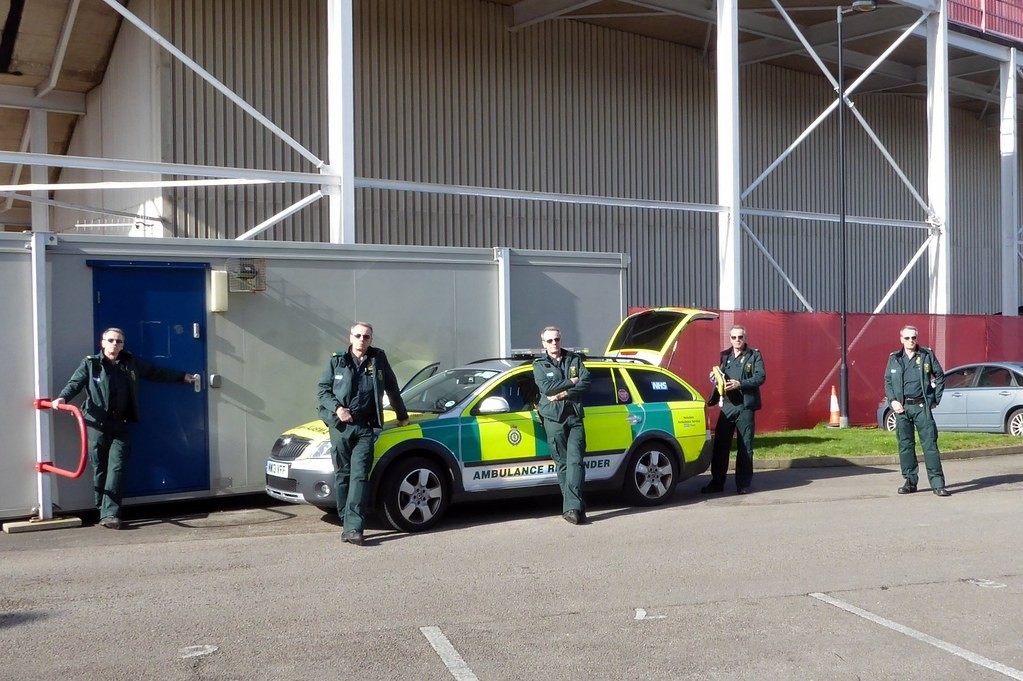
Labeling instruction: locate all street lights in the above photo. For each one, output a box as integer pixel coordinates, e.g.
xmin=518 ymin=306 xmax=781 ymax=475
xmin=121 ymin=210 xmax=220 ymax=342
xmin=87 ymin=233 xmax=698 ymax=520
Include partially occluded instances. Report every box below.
xmin=837 ymin=1 xmax=878 ymax=427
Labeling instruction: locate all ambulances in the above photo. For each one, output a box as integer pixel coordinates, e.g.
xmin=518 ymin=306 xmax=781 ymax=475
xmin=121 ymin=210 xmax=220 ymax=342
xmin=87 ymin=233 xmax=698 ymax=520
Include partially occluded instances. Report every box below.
xmin=265 ymin=306 xmax=719 ymax=534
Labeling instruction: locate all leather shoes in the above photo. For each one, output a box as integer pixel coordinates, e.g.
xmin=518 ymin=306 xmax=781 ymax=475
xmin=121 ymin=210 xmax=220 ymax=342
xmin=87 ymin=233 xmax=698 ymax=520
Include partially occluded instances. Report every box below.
xmin=897 ymin=484 xmax=917 ymax=493
xmin=933 ymin=487 xmax=949 ymax=495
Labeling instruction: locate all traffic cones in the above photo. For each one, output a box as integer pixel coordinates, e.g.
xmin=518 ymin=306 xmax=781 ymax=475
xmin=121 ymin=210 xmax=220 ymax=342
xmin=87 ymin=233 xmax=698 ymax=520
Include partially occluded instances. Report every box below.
xmin=826 ymin=385 xmax=841 ymax=427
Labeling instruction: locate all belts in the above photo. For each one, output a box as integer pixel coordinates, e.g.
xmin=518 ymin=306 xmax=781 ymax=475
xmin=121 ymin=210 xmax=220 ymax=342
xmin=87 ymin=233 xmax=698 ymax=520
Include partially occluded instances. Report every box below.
xmin=723 ymin=398 xmax=739 ymax=403
xmin=904 ymin=399 xmax=925 ymax=405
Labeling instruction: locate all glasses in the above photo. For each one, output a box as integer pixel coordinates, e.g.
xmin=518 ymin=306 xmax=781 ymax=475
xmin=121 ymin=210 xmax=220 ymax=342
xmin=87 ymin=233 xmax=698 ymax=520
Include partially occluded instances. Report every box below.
xmin=544 ymin=338 xmax=560 ymax=343
xmin=351 ymin=334 xmax=370 ymax=339
xmin=902 ymin=336 xmax=916 ymax=340
xmin=103 ymin=338 xmax=124 ymax=344
xmin=730 ymin=336 xmax=743 ymax=339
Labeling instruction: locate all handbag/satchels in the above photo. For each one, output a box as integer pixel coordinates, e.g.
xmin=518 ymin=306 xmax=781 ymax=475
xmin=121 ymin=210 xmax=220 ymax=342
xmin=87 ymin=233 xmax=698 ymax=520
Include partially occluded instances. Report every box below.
xmin=712 ymin=366 xmax=727 ymax=397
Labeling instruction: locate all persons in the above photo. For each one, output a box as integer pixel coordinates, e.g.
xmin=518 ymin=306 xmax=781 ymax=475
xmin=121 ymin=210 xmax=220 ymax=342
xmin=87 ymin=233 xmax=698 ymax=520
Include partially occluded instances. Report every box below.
xmin=316 ymin=322 xmax=410 ymax=544
xmin=884 ymin=326 xmax=950 ymax=496
xmin=699 ymin=326 xmax=766 ymax=493
xmin=533 ymin=327 xmax=591 ymax=524
xmin=52 ymin=328 xmax=196 ymax=529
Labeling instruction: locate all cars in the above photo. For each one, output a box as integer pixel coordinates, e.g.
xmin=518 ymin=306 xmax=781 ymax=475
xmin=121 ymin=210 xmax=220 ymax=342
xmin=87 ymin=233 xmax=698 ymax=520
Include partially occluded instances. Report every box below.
xmin=878 ymin=362 xmax=1022 ymax=436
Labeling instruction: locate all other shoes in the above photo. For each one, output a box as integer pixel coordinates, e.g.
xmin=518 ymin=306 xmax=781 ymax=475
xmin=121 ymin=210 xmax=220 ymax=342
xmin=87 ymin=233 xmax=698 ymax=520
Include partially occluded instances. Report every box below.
xmin=341 ymin=530 xmax=364 ymax=545
xmin=99 ymin=516 xmax=123 ymax=530
xmin=737 ymin=486 xmax=750 ymax=494
xmin=579 ymin=509 xmax=588 ymax=522
xmin=701 ymin=484 xmax=724 ymax=493
xmin=562 ymin=510 xmax=580 ymax=524
